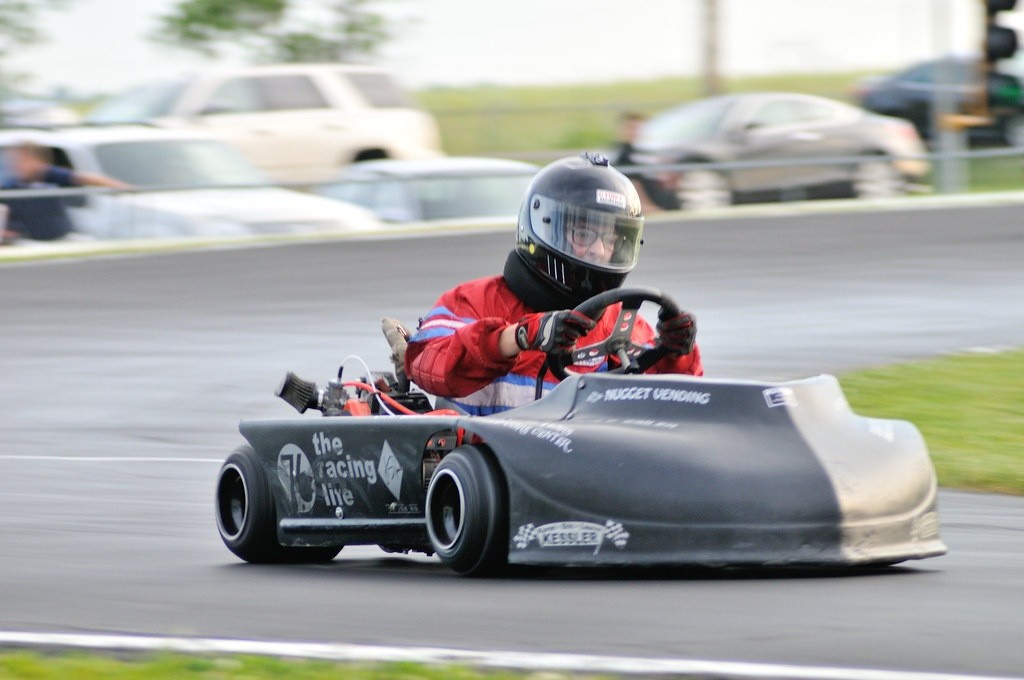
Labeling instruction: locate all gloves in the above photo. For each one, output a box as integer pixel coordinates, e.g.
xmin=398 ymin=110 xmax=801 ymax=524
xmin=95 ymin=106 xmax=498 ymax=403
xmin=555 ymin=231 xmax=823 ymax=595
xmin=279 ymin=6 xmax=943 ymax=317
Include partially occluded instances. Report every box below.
xmin=513 ymin=308 xmax=597 ymax=358
xmin=654 ymin=311 xmax=698 ymax=355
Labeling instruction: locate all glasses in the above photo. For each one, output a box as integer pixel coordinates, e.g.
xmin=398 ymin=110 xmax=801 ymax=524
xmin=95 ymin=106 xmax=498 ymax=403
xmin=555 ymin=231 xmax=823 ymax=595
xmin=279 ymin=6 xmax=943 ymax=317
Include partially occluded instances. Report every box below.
xmin=563 ymin=225 xmax=627 ymax=253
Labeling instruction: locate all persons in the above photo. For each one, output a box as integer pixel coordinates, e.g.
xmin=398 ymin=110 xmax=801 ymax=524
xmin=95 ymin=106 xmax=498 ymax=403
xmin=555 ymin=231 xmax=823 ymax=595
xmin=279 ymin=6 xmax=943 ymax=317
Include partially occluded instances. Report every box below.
xmin=404 ymin=152 xmax=704 ymax=418
xmin=614 ymin=114 xmax=680 ymax=213
xmin=0 ymin=144 xmax=133 ymax=247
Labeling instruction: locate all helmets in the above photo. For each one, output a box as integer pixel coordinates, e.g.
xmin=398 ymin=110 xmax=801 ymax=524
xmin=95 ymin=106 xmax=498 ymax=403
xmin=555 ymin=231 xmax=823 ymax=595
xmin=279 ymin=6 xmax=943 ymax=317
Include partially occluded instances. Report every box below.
xmin=513 ymin=149 xmax=646 ymax=307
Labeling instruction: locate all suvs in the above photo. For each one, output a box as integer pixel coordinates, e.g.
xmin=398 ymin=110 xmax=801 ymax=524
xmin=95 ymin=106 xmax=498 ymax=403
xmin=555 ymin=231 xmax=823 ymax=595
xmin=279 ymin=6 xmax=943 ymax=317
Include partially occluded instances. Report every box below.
xmin=0 ymin=118 xmax=382 ymax=245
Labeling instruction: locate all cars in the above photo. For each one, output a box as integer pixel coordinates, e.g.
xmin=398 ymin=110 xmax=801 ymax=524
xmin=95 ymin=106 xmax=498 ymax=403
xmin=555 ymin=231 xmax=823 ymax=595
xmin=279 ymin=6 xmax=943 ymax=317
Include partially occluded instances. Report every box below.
xmin=142 ymin=64 xmax=444 ymax=192
xmin=312 ymin=156 xmax=546 ymax=222
xmin=860 ymin=52 xmax=1024 ymax=150
xmin=629 ymin=90 xmax=931 ymax=212
